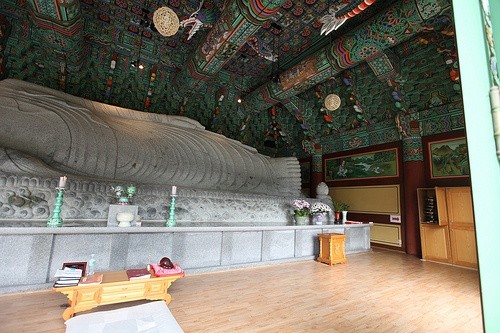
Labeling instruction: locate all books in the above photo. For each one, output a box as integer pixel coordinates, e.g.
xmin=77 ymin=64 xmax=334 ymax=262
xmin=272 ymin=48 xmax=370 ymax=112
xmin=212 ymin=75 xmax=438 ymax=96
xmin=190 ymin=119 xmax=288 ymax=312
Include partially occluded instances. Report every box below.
xmin=126 ymin=268 xmax=151 ymax=281
xmin=78 ymin=273 xmax=104 ymax=286
xmin=54 ymin=266 xmax=83 ymax=287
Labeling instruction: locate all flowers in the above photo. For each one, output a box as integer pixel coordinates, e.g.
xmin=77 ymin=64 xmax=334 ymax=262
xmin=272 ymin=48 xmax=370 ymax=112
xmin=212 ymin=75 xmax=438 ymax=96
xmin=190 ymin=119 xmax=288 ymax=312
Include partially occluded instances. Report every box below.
xmin=293 ymin=199 xmax=310 ymax=215
xmin=309 ymin=202 xmax=332 ymax=215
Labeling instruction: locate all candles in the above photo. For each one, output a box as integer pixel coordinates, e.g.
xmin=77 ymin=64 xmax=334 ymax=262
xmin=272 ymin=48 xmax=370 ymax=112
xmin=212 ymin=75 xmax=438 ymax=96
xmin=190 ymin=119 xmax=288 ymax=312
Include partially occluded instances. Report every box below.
xmin=59 ymin=175 xmax=67 ymax=188
xmin=172 ymin=185 xmax=177 ymax=195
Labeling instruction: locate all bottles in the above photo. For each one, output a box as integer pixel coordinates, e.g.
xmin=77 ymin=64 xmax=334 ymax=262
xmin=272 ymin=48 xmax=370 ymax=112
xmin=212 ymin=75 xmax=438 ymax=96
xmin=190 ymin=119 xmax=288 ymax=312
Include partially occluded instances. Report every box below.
xmin=88 ymin=253 xmax=95 ymax=275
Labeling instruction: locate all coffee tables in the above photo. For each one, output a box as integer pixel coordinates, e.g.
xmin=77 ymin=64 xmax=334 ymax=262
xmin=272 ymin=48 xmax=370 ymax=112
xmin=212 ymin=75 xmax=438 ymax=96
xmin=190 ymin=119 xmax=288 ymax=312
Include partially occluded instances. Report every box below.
xmin=51 ymin=262 xmax=185 ymax=322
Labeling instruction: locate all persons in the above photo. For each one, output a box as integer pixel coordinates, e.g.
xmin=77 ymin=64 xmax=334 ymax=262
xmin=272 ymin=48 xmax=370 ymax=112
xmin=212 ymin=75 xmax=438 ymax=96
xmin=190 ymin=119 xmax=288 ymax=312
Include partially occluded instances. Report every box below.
xmin=0 ymin=78 xmax=302 ymax=197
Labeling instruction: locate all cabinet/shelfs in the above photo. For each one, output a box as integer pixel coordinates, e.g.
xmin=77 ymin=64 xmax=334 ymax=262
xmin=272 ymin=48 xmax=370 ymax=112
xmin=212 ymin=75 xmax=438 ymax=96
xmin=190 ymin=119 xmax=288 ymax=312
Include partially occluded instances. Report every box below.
xmin=416 ymin=187 xmax=478 ymax=266
xmin=316 ymin=232 xmax=346 ymax=264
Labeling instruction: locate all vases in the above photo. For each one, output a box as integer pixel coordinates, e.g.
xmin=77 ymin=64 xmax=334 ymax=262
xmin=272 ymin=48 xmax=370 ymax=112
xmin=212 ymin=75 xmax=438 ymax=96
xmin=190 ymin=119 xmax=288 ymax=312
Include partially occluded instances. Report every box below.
xmin=293 ymin=215 xmax=309 ymax=225
xmin=312 ymin=212 xmax=327 ymax=225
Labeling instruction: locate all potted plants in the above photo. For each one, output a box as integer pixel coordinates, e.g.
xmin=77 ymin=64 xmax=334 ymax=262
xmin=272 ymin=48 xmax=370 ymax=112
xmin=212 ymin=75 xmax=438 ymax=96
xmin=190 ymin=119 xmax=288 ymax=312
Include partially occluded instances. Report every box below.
xmin=341 ymin=201 xmax=350 ymax=224
xmin=333 ymin=201 xmax=343 ymax=224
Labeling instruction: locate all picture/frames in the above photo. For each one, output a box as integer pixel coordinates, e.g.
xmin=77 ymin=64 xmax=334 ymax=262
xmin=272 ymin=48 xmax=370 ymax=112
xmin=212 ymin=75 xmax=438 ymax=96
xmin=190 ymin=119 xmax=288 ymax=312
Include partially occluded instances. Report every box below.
xmin=421 ymin=127 xmax=470 ymax=187
xmin=322 ymin=139 xmax=404 ymax=184
